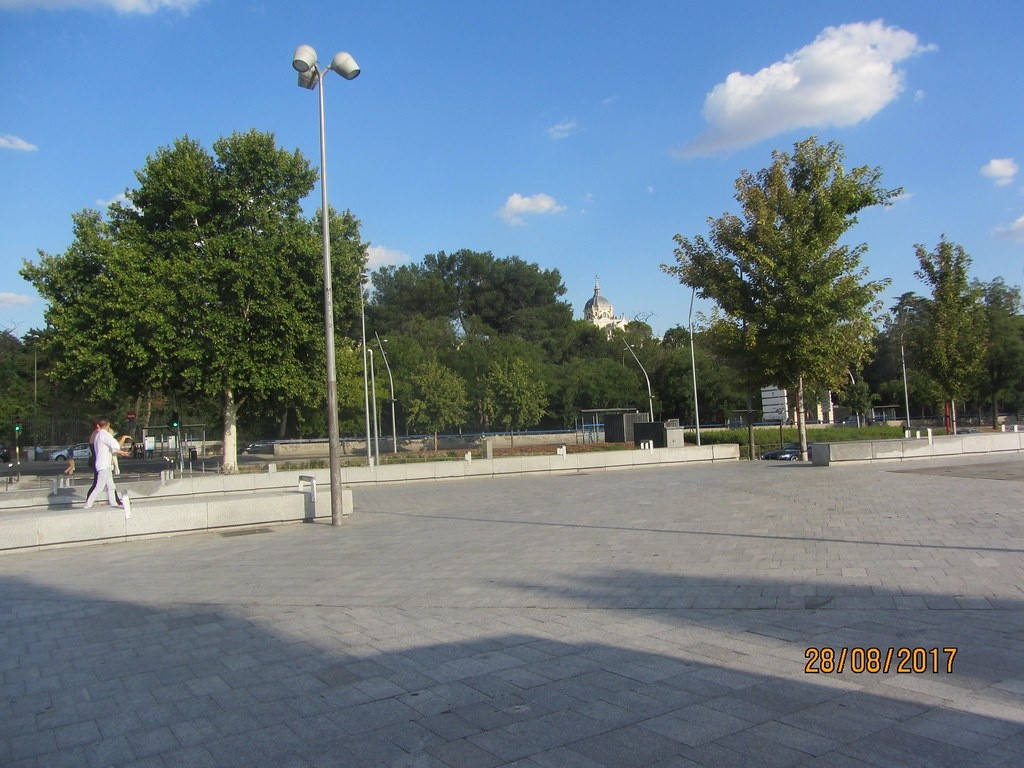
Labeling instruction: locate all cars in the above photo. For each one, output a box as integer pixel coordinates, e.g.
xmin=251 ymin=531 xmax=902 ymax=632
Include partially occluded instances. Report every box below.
xmin=48 ymin=443 xmax=92 ymax=462
xmin=834 ymin=415 xmax=859 ymax=423
xmin=760 ymin=442 xmax=813 ymax=461
xmin=240 ymin=439 xmax=275 ymax=455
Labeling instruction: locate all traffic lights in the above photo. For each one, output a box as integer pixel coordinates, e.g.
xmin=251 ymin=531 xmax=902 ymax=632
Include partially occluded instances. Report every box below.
xmin=171 ymin=420 xmax=178 ymax=428
xmin=13 ymin=415 xmax=21 ymax=431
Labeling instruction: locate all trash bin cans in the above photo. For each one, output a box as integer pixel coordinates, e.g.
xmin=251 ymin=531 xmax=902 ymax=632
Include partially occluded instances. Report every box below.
xmin=191 ymin=449 xmax=197 ymax=461
xmin=811 ymin=442 xmax=831 ymax=467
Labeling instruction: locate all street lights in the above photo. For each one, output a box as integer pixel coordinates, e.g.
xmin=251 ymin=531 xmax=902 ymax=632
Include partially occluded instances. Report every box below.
xmin=293 ymin=44 xmax=360 ymax=526
xmin=623 ymin=345 xmax=634 ymax=367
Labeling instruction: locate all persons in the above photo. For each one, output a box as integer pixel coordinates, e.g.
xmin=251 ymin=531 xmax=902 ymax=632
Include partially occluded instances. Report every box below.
xmin=83 ymin=419 xmax=132 ymax=508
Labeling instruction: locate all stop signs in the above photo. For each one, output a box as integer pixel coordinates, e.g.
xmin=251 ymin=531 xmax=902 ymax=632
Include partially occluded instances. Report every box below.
xmin=127 ymin=411 xmax=136 ymax=420
